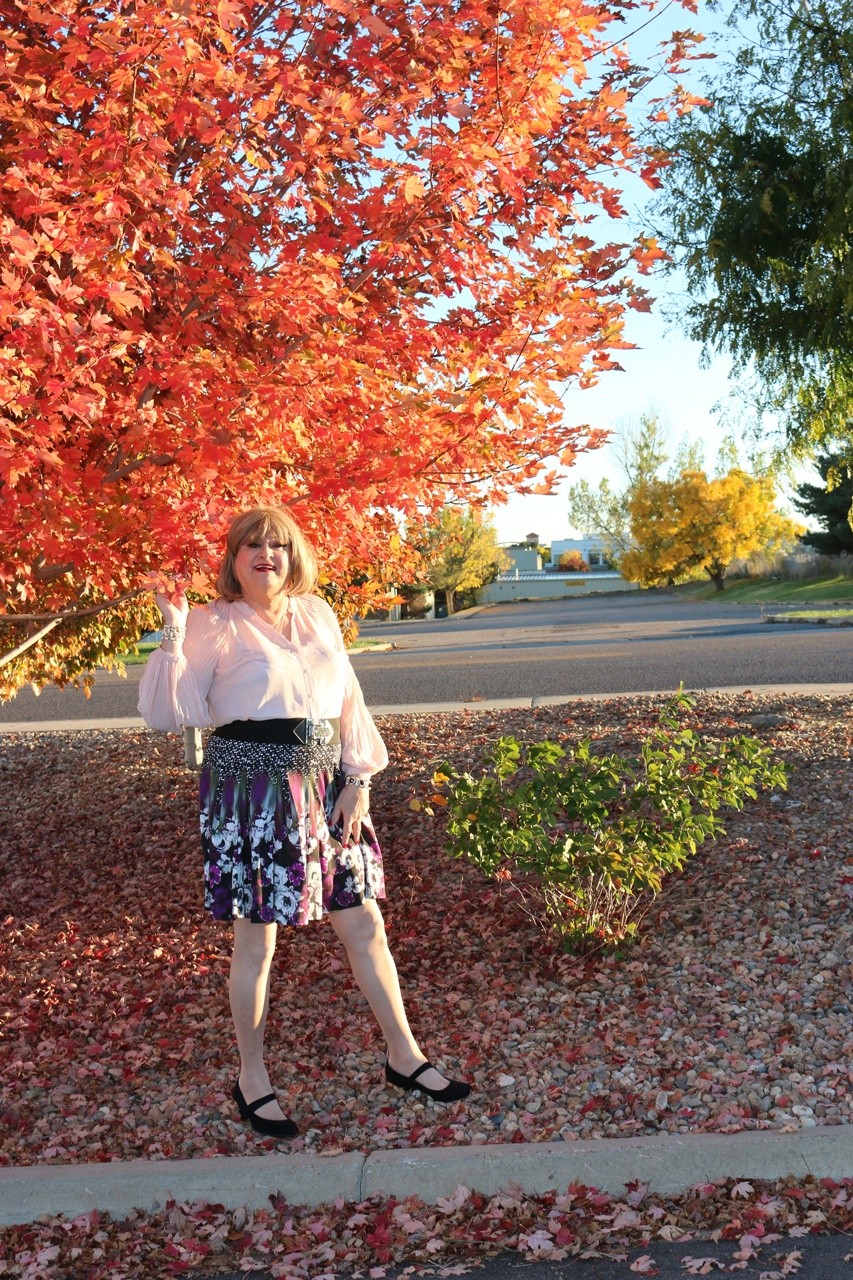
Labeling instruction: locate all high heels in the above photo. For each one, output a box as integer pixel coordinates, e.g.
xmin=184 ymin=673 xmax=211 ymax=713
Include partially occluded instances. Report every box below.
xmin=232 ymin=1075 xmax=299 ymax=1137
xmin=385 ymin=1057 xmax=470 ymax=1101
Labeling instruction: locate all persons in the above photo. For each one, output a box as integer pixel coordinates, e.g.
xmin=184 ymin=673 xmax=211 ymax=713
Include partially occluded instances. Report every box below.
xmin=136 ymin=504 xmax=475 ymax=1138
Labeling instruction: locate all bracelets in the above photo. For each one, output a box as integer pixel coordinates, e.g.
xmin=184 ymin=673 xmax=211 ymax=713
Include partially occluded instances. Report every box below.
xmin=160 ymin=624 xmax=188 ymax=643
xmin=345 ymin=775 xmax=373 ymax=791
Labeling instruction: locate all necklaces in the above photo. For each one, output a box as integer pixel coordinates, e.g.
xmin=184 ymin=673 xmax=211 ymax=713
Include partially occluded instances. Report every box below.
xmin=278 ymin=595 xmax=288 ymax=636
